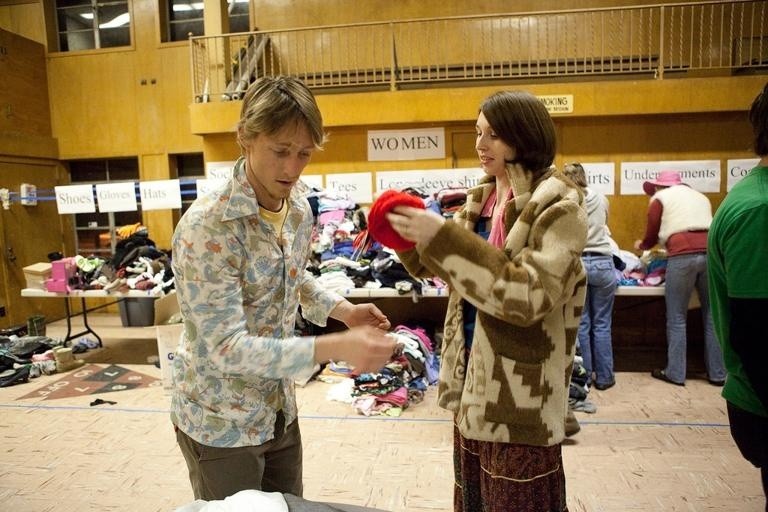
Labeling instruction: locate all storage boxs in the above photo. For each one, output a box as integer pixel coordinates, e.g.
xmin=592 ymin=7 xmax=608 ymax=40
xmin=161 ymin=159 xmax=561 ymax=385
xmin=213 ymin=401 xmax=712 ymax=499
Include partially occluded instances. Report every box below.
xmin=23 ymin=261 xmax=52 ymax=287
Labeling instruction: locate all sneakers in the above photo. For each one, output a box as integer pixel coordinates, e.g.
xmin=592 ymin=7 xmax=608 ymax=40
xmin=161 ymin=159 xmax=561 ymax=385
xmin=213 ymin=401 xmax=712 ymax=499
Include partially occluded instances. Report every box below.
xmin=652 ymin=368 xmax=684 ymax=385
xmin=708 ymin=380 xmax=724 ymax=385
xmin=587 ymin=379 xmax=615 ymax=390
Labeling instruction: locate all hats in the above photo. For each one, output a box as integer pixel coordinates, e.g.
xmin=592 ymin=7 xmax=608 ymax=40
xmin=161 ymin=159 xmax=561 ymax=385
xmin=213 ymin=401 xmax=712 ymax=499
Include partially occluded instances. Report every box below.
xmin=643 ymin=171 xmax=682 ymax=196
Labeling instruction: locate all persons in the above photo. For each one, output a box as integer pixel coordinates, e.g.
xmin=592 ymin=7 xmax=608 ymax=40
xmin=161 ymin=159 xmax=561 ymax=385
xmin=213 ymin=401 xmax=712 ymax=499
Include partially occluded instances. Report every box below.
xmin=167 ymin=72 xmax=397 ymax=501
xmin=386 ymin=89 xmax=589 ymax=512
xmin=632 ymin=171 xmax=726 ymax=387
xmin=706 ymin=90 xmax=767 ymax=512
xmin=561 ymin=161 xmax=616 ymax=390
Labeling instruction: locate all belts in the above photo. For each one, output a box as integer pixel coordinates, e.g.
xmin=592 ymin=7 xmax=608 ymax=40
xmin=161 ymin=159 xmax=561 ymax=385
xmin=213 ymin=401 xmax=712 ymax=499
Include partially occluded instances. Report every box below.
xmin=583 ymin=252 xmax=600 ymax=256
